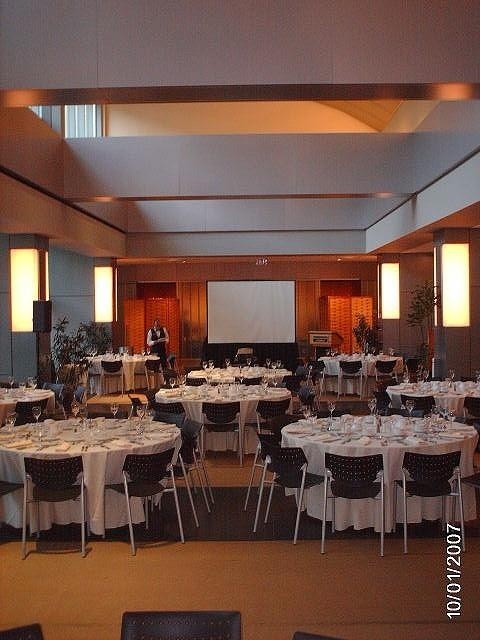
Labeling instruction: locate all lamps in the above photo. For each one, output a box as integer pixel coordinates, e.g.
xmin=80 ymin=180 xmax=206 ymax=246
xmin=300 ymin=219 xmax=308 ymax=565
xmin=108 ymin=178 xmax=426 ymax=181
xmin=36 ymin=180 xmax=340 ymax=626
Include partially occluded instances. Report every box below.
xmin=375 ymin=252 xmax=401 ymax=320
xmin=92 ymin=257 xmax=118 ymax=323
xmin=6 ymin=233 xmax=50 ymax=333
xmin=431 ymin=227 xmax=471 ymax=327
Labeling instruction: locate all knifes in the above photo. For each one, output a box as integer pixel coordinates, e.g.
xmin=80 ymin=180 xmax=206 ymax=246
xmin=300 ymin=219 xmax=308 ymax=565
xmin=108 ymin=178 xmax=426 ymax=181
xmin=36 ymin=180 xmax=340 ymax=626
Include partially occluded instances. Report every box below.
xmin=397 ymin=438 xmax=410 ymax=449
xmin=341 ymin=437 xmax=351 ymax=445
xmin=81 ymin=444 xmax=89 ymax=454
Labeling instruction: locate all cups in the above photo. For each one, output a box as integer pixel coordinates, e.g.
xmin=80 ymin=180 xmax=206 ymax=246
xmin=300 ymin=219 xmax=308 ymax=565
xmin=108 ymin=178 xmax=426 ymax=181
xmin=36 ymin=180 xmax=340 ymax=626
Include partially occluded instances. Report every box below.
xmin=277 ymin=359 xmax=281 ymax=369
xmin=371 ymin=346 xmax=376 ymax=356
xmin=325 ymin=349 xmax=330 ymax=356
xmin=31 ymin=406 xmax=41 ymax=424
xmin=423 ymin=369 xmax=429 ymax=383
xmin=367 ymin=398 xmax=376 ymax=416
xmin=169 ymin=378 xmax=175 ymax=391
xmin=137 ymin=405 xmax=145 ymax=424
xmin=447 ymin=409 xmax=456 ymax=427
xmin=405 ymin=400 xmax=415 ymax=419
xmin=110 ymin=401 xmax=119 ymax=418
xmin=308 ymin=413 xmax=318 ymax=435
xmin=302 ymin=404 xmax=312 ymax=423
xmin=331 ymin=350 xmax=335 ymax=358
xmin=238 ymin=374 xmax=245 ymax=385
xmin=246 ymin=357 xmax=252 ymax=368
xmin=19 ymin=382 xmax=26 ymax=393
xmin=8 ymin=376 xmax=15 ymax=389
xmin=27 ymin=376 xmax=33 ymax=389
xmin=202 ymin=361 xmax=207 ymax=370
xmin=6 ymin=417 xmax=15 ymax=430
xmin=266 ymin=358 xmax=271 ymax=369
xmin=326 ymin=399 xmax=336 ymax=419
xmin=31 ymin=380 xmax=37 ymax=389
xmin=224 ymin=357 xmax=230 ymax=367
xmin=180 ymin=374 xmax=187 ymax=383
xmin=147 ymin=347 xmax=151 ymax=354
xmin=261 ymin=377 xmax=268 ymax=388
xmin=210 ymin=359 xmax=215 ymax=370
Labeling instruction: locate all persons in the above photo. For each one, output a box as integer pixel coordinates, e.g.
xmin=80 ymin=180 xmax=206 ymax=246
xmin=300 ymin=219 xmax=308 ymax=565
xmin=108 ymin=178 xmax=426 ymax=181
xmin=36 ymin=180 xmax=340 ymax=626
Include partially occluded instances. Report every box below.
xmin=147 ymin=319 xmax=169 ymax=373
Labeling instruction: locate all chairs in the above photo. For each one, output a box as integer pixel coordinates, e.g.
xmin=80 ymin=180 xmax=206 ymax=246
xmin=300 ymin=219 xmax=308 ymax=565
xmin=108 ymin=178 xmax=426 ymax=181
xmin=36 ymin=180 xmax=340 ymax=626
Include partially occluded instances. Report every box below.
xmin=238 ymin=398 xmax=291 ymax=467
xmin=105 ymin=448 xmax=185 ymax=555
xmin=380 ymin=408 xmax=410 ymax=417
xmin=317 ymin=408 xmax=353 ymax=416
xmin=253 ymin=441 xmax=329 ymax=545
xmin=400 ymin=394 xmax=436 ymax=417
xmin=101 ymin=360 xmax=124 ymax=394
xmin=152 ymin=399 xmax=201 ymax=453
xmin=462 ymin=397 xmax=480 ymax=423
xmin=182 ymin=419 xmax=215 ymax=507
xmin=43 ymin=382 xmax=66 ymax=420
xmin=201 ymin=402 xmax=243 ymax=467
xmin=307 ymin=360 xmax=339 ymax=399
xmin=282 ymin=373 xmax=306 ymax=405
xmin=2 ymin=623 xmax=43 ymax=639
xmin=271 ymin=415 xmax=305 ymax=445
xmin=393 ymin=451 xmax=466 ymax=553
xmin=0 ymin=481 xmax=24 ymax=497
xmin=320 ymin=452 xmax=386 ymax=558
xmin=135 ymin=360 xmax=161 ymax=392
xmin=185 ymin=377 xmax=207 ymax=386
xmin=364 ymin=360 xmax=398 ymax=398
xmin=120 ymin=610 xmax=243 ymax=640
xmin=242 ymin=377 xmax=262 ymax=385
xmin=245 ymin=426 xmax=282 ymax=514
xmin=165 ymin=439 xmax=212 ymax=529
xmin=452 ymin=473 xmax=480 ymax=529
xmin=373 ymin=391 xmax=393 ymax=415
xmin=22 ymin=456 xmax=88 ymax=558
xmin=15 ymin=397 xmax=48 ymax=416
xmin=293 ymin=629 xmax=341 ymax=640
xmin=339 ymin=360 xmax=362 ymax=399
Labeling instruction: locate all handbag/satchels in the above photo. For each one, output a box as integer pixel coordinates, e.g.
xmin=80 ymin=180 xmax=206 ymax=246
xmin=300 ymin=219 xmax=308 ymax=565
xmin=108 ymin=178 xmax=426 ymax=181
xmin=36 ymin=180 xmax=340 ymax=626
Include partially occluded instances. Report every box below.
xmin=2 ymin=439 xmax=32 ymax=448
xmin=55 ymin=442 xmax=71 ymax=452
xmin=111 ymin=440 xmax=132 ymax=447
xmin=146 ymin=431 xmax=173 ymax=438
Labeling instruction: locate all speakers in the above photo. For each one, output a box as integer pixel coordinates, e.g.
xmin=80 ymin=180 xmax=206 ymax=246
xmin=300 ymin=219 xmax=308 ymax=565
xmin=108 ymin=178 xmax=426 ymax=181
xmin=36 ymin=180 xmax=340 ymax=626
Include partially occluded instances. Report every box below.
xmin=32 ymin=299 xmax=52 ymax=334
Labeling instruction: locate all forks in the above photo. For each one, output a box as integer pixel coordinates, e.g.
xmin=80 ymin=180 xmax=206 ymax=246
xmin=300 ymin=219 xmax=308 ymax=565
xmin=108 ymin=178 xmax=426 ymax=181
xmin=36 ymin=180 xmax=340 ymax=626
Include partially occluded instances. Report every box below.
xmin=297 ymin=432 xmax=317 ymax=440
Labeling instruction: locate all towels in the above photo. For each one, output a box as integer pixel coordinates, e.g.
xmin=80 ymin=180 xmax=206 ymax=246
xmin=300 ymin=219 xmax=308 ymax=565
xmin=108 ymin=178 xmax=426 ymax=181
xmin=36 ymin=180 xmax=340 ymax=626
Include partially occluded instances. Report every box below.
xmin=438 ymin=432 xmax=467 ymax=439
xmin=321 ymin=437 xmax=341 ymax=442
xmin=358 ymin=436 xmax=371 ymax=446
xmin=407 ymin=437 xmax=427 ymax=445
xmin=454 ymin=425 xmax=474 ymax=431
